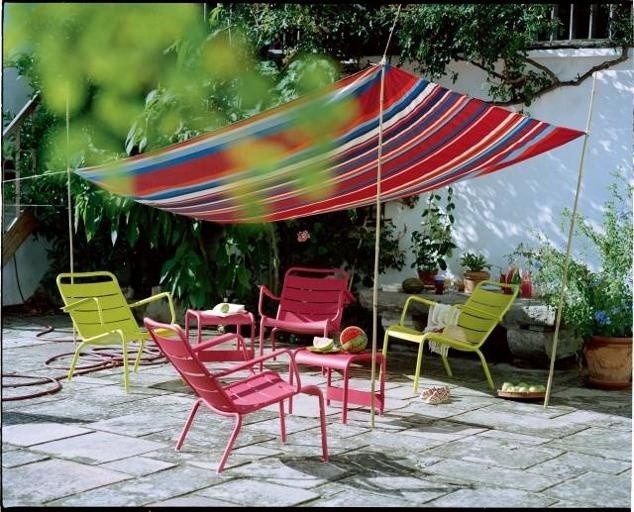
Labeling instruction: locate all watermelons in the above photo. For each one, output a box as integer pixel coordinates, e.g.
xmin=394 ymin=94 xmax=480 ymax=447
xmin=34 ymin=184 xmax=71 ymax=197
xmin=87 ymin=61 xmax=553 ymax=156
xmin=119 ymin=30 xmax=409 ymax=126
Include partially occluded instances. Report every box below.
xmin=312 ymin=336 xmax=334 ymax=352
xmin=339 ymin=326 xmax=368 ymax=353
xmin=403 ymin=278 xmax=425 ymax=293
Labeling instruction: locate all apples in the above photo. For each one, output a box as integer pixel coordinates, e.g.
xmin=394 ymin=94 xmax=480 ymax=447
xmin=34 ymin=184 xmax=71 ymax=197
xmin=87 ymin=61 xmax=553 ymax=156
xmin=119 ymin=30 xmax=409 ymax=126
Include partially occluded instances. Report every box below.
xmin=501 ymin=381 xmax=545 ymax=394
xmin=221 ymin=304 xmax=229 ymax=313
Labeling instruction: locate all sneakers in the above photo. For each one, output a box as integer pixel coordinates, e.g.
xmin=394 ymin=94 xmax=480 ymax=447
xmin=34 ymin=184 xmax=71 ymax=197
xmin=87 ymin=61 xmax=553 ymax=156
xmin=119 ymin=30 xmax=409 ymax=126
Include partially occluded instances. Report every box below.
xmin=421 ymin=387 xmax=449 ymax=405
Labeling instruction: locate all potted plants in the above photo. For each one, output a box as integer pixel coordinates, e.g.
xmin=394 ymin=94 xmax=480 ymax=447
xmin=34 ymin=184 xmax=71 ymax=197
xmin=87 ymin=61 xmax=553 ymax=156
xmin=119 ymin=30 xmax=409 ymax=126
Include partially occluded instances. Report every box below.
xmin=410 ymin=187 xmax=457 ymax=285
xmin=507 ymin=172 xmax=633 ymax=387
xmin=460 ymin=251 xmax=494 ymax=294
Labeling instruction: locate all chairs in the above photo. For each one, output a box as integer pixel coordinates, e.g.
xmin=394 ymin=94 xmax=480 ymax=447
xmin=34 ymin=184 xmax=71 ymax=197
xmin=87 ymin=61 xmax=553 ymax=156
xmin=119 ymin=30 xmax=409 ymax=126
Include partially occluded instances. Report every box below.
xmin=55 ymin=267 xmax=520 ymax=474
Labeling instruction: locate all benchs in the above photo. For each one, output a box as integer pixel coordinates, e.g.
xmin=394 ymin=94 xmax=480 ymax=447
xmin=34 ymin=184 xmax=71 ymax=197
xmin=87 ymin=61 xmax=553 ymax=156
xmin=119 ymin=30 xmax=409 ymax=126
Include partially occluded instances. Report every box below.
xmin=358 ymin=282 xmax=585 ymax=366
